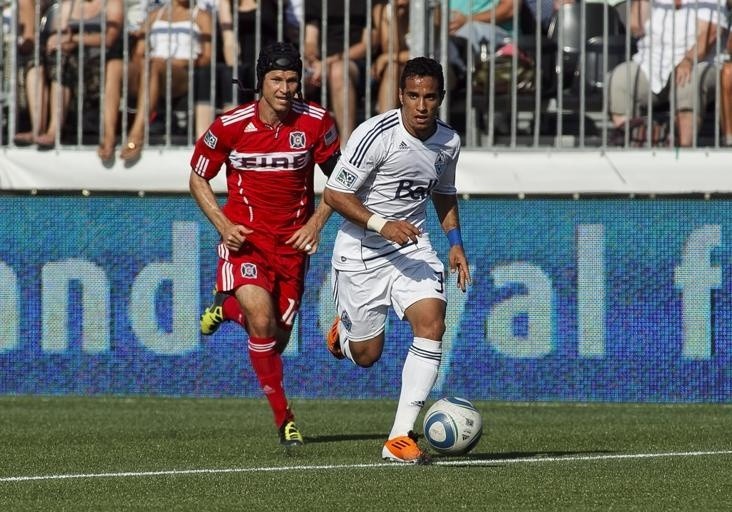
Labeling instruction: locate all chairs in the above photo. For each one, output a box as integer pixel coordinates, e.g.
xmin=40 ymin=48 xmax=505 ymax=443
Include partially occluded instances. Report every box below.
xmin=543 ymin=6 xmax=636 ymax=112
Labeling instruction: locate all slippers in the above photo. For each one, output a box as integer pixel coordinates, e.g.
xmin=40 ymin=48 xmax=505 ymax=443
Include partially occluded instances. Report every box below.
xmin=98 ymin=138 xmax=144 ymax=162
xmin=14 ymin=132 xmax=55 ymax=148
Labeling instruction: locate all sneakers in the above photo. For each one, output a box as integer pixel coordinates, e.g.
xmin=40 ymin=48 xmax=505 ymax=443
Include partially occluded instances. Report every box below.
xmin=278 ymin=421 xmax=304 ymax=449
xmin=199 ymin=287 xmax=230 ymax=337
xmin=382 ymin=435 xmax=432 ymax=465
xmin=326 ymin=316 xmax=345 ymax=361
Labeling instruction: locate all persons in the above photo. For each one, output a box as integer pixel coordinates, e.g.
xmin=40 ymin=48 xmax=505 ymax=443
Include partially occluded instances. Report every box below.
xmin=317 ymin=52 xmax=473 ymax=468
xmin=177 ymin=39 xmax=347 ymax=449
xmin=0 ymin=1 xmax=728 ymax=167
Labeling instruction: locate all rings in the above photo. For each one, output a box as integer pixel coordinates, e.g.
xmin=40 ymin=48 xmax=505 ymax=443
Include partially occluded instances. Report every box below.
xmin=305 ymin=243 xmax=312 ymax=251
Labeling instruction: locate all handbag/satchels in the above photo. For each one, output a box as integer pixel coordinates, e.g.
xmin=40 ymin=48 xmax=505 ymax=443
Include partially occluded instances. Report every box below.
xmin=606 ymin=114 xmax=673 ymax=149
xmin=471 ymin=44 xmax=547 ymax=94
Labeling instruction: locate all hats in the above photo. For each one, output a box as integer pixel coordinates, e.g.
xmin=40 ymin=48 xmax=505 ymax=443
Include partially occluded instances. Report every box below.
xmin=253 ymin=42 xmax=303 ymax=94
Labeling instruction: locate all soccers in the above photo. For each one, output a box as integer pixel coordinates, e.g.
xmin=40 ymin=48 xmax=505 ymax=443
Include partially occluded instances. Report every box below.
xmin=423 ymin=396 xmax=482 ymax=457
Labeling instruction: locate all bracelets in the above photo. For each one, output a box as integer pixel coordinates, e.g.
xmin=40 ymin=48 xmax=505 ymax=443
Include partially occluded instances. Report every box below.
xmin=444 ymin=226 xmax=464 ymax=247
xmin=366 ymin=213 xmax=390 ymax=234
